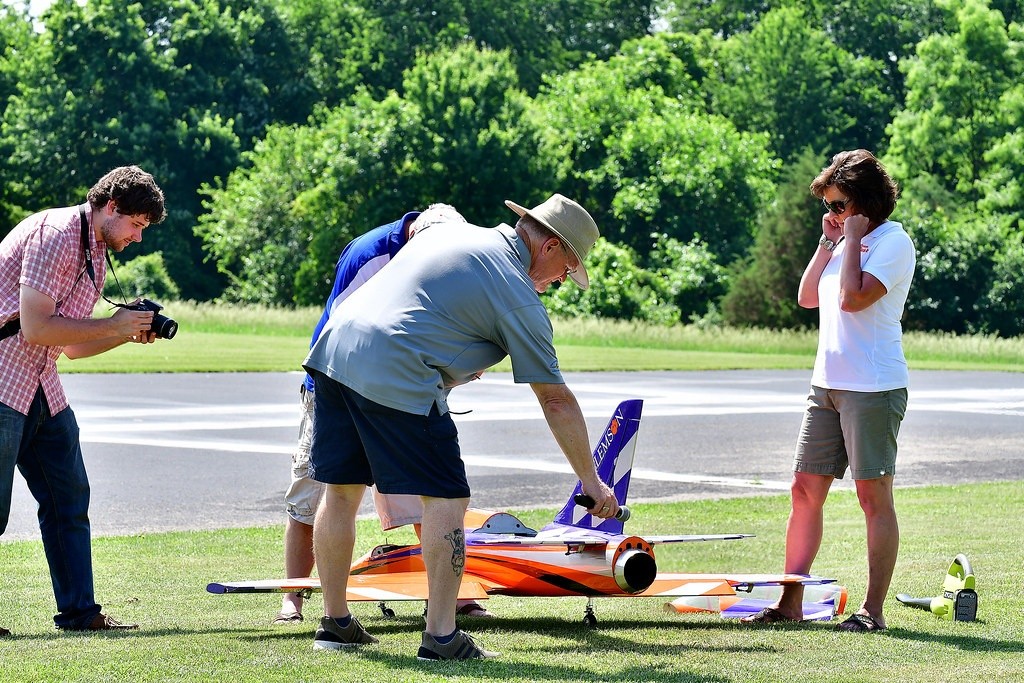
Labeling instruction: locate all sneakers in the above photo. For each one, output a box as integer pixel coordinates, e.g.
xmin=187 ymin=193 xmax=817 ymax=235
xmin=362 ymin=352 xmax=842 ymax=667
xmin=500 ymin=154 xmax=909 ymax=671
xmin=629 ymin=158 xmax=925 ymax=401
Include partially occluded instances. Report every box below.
xmin=313 ymin=615 xmax=380 ymax=651
xmin=417 ymin=632 xmax=503 ymax=662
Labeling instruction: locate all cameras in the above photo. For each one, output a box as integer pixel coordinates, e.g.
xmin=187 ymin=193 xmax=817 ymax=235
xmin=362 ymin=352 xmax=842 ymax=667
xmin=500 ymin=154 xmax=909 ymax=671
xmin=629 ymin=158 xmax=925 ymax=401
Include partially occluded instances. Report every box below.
xmin=133 ymin=298 xmax=178 ymax=341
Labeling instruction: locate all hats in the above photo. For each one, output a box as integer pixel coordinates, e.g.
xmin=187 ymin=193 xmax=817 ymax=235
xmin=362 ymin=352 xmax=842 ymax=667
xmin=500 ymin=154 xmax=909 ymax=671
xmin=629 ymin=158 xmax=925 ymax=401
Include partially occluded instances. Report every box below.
xmin=505 ymin=194 xmax=600 ymax=291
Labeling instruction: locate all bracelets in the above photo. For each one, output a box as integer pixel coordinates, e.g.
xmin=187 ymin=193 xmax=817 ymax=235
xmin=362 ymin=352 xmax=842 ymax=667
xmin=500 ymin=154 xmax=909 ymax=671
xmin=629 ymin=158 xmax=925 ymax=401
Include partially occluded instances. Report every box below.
xmin=819 ymin=233 xmax=837 ymax=252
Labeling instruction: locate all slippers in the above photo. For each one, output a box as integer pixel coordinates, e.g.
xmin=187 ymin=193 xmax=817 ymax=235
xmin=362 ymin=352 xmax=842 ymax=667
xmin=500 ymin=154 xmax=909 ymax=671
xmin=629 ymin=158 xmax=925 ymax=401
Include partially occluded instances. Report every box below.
xmin=456 ymin=604 xmax=486 ymax=615
xmin=841 ymin=614 xmax=883 ymax=633
xmin=749 ymin=608 xmax=801 ymax=625
xmin=273 ymin=612 xmax=304 ymax=623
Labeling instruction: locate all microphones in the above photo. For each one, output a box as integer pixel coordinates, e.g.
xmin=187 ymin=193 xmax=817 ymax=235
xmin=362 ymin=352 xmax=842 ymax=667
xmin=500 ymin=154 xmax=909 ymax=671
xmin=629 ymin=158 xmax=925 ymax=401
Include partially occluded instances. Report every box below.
xmin=573 ymin=493 xmax=631 ymax=523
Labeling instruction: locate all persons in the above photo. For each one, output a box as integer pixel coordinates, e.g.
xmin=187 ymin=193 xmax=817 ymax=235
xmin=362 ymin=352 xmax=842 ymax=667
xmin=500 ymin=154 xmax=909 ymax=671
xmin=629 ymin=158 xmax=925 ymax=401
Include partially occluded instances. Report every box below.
xmin=302 ymin=192 xmax=619 ymax=663
xmin=0 ymin=165 xmax=165 ymax=635
xmin=271 ymin=203 xmax=497 ymax=627
xmin=739 ymin=149 xmax=919 ymax=630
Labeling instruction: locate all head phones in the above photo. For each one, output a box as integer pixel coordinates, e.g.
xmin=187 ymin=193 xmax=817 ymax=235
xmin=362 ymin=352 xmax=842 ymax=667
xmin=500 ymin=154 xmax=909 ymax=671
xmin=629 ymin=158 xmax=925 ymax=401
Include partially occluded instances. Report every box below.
xmin=929 ymin=554 xmax=974 ymax=621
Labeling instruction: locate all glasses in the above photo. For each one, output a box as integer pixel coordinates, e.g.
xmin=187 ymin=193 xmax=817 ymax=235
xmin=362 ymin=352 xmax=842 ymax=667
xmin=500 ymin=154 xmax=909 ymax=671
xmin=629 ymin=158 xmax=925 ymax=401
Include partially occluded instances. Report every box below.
xmin=823 ymin=197 xmax=852 ymax=214
xmin=555 ymin=237 xmax=577 ymax=274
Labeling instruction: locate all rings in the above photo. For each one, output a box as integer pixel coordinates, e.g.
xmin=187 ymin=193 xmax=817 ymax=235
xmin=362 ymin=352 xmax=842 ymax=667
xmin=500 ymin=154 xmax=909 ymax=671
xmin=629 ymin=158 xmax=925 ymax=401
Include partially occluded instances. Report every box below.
xmin=603 ymin=506 xmax=610 ymax=512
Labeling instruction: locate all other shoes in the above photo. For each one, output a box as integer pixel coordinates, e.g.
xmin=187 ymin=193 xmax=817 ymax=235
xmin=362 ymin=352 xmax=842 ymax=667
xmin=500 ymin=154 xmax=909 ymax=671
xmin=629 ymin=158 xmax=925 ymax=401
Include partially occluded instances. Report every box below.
xmin=0 ymin=627 xmax=9 ymax=636
xmin=58 ymin=612 xmax=139 ymax=631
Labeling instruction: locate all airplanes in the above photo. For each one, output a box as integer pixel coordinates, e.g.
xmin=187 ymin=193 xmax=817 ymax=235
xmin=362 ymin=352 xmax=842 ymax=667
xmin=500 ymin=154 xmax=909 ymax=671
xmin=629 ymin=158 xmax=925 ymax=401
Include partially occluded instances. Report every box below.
xmin=206 ymin=399 xmax=839 ymax=630
